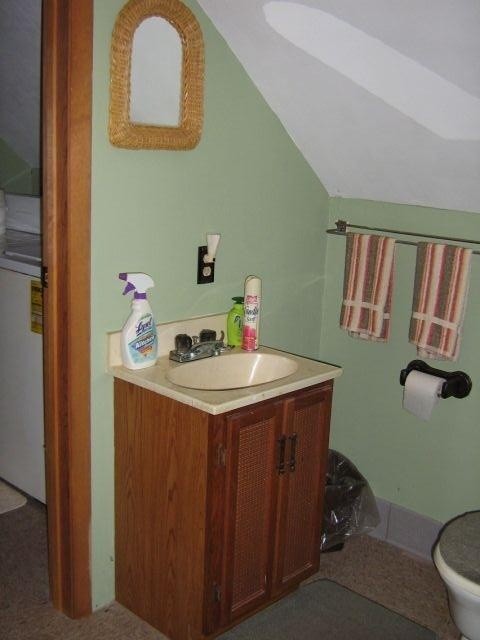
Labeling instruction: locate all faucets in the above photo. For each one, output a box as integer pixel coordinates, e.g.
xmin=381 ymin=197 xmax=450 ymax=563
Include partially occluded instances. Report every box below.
xmin=189 ymin=341 xmax=223 ymax=358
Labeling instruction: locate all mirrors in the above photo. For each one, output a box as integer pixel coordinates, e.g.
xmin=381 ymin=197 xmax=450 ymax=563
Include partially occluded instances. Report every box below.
xmin=108 ymin=0 xmax=206 ymax=151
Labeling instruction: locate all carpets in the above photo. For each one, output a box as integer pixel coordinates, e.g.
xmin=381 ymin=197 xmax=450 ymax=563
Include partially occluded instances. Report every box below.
xmin=213 ymin=579 xmax=437 ymax=640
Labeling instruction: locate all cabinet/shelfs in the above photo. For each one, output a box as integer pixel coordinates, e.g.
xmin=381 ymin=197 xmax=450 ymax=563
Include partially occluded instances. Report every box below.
xmin=114 ymin=377 xmax=335 ymax=640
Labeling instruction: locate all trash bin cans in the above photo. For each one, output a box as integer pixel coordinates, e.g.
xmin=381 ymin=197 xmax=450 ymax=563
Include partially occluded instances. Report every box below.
xmin=322 ymin=450 xmax=366 ymax=552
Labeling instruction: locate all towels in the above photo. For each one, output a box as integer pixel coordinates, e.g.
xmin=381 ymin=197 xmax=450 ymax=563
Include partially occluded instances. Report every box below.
xmin=339 ymin=231 xmax=473 ymax=363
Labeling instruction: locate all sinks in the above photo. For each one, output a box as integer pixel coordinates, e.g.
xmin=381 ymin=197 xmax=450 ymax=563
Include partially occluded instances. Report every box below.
xmin=167 ymin=352 xmax=298 ymax=391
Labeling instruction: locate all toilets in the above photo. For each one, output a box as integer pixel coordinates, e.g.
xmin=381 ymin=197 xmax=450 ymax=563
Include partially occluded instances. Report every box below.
xmin=433 ymin=510 xmax=480 ymax=640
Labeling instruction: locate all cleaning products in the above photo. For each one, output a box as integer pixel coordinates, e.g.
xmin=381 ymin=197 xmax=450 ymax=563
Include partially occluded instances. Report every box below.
xmin=118 ymin=272 xmax=158 ymax=370
